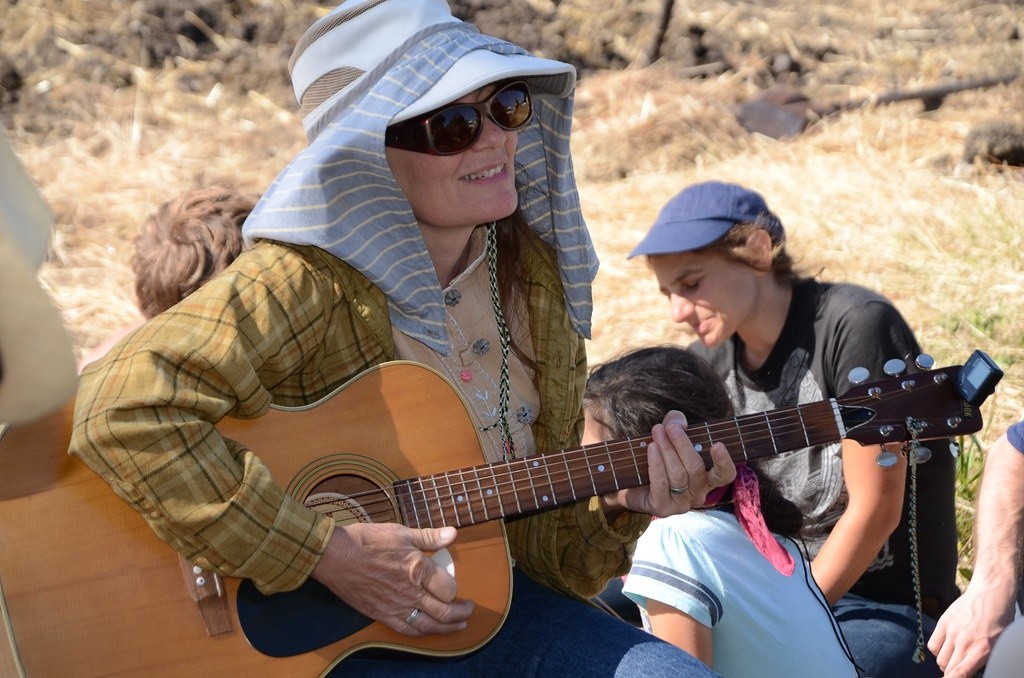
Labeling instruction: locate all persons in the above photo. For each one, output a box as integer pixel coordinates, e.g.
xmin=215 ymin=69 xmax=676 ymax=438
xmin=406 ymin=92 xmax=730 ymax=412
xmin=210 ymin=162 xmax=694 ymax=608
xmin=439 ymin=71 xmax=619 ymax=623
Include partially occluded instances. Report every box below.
xmin=69 ymin=0 xmax=740 ymax=678
xmin=132 ymin=186 xmax=254 ymax=321
xmin=583 ymin=181 xmax=1024 ymax=678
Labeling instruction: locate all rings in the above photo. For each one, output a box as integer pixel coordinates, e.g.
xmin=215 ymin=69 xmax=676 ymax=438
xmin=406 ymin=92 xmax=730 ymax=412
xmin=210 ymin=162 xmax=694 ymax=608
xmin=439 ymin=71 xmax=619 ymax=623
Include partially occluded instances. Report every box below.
xmin=669 ymin=485 xmax=688 ymax=496
xmin=407 ymin=608 xmax=421 ymax=625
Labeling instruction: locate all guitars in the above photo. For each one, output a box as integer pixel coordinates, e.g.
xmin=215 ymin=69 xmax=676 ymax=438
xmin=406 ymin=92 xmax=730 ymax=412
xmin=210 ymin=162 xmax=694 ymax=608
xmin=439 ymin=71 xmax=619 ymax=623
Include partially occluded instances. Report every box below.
xmin=0 ymin=358 xmax=986 ymax=678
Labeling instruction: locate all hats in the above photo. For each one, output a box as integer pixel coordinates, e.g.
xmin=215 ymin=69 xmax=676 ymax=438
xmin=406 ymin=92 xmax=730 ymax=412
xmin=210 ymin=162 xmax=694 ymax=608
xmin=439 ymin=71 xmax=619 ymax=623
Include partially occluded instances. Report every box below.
xmin=241 ymin=1 xmax=600 ymax=356
xmin=623 ymin=182 xmax=785 ymax=259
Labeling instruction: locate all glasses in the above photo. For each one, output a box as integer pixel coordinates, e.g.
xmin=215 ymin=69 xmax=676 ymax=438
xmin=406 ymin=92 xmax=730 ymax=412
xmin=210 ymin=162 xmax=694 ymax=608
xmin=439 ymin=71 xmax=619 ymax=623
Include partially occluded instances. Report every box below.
xmin=384 ymin=82 xmax=533 ymax=155
